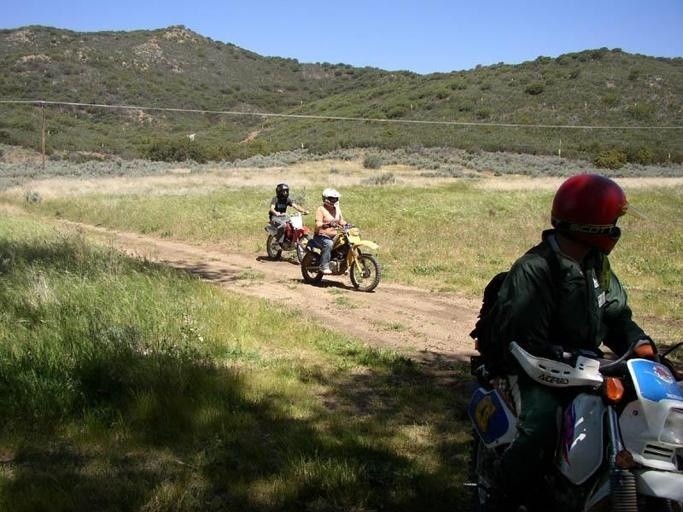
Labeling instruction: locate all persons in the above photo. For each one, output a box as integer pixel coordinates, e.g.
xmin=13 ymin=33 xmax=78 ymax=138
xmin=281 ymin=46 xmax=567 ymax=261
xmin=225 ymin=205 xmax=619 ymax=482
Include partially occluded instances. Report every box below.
xmin=476 ymin=174 xmax=679 ymax=512
xmin=268 ymin=184 xmax=309 ymax=248
xmin=314 ymin=188 xmax=353 ymax=275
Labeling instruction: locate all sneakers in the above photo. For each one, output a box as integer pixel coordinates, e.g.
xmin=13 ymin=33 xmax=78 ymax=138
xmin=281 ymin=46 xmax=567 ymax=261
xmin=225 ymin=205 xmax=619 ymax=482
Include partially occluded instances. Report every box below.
xmin=319 ymin=266 xmax=333 ymax=275
xmin=272 ymin=242 xmax=281 ymax=251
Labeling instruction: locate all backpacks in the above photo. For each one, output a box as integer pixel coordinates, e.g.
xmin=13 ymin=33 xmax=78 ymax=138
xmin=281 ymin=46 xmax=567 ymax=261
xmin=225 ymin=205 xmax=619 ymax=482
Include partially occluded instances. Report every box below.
xmin=469 ymin=247 xmax=560 ymax=379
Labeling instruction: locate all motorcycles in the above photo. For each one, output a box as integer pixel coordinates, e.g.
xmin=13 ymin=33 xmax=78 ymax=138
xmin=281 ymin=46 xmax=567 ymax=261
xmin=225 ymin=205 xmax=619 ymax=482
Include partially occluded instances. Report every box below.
xmin=466 ymin=325 xmax=683 ymax=512
xmin=264 ymin=218 xmax=380 ymax=292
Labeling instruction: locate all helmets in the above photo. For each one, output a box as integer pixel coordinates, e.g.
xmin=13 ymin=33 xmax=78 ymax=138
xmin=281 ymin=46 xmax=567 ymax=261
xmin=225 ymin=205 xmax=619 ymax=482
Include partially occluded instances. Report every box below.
xmin=276 ymin=184 xmax=289 ymax=198
xmin=550 ymin=174 xmax=628 ymax=255
xmin=322 ymin=189 xmax=341 ymax=208
